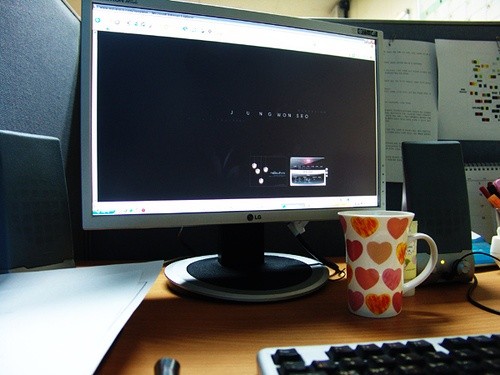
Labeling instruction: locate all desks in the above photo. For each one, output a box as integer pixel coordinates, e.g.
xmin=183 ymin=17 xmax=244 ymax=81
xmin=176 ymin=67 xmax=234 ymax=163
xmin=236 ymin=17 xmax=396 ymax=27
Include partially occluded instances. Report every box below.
xmin=93 ymin=257 xmax=500 ymax=374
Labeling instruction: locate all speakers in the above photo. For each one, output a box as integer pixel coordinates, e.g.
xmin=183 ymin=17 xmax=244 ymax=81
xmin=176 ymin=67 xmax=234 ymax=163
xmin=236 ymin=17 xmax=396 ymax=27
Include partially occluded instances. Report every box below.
xmin=400 ymin=140 xmax=476 ymax=289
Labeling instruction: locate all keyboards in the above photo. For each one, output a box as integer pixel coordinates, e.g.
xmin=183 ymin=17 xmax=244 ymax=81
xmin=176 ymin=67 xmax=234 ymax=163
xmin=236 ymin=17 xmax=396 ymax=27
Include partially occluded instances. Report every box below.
xmin=256 ymin=333 xmax=499 ymax=375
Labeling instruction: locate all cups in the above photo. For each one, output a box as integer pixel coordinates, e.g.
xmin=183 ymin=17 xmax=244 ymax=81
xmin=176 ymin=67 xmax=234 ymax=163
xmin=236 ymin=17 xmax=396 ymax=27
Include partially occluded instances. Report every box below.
xmin=337 ymin=209 xmax=438 ymax=319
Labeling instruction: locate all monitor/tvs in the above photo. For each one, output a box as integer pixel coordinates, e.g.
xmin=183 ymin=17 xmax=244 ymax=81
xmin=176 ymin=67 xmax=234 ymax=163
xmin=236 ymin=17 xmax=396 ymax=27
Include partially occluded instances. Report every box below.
xmin=80 ymin=0 xmax=387 ymax=305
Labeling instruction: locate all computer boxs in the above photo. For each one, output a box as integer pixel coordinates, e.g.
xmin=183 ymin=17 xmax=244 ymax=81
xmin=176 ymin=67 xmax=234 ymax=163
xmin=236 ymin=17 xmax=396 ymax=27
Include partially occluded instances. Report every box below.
xmin=0 ymin=0 xmax=153 ymax=271
